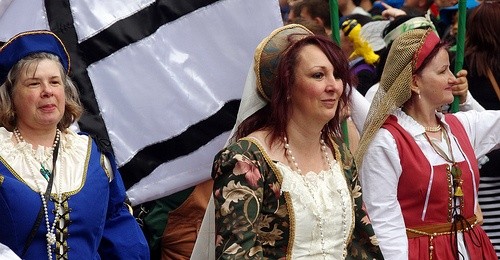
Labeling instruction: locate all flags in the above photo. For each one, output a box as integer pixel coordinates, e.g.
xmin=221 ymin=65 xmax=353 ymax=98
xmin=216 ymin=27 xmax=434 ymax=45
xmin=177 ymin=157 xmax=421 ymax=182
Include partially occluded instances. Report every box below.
xmin=0 ymin=0 xmax=283 ymax=206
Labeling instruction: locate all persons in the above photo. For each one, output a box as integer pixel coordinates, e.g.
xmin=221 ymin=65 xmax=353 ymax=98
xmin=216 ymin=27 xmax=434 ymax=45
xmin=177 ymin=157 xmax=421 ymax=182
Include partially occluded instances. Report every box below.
xmin=132 ymin=0 xmax=500 ymax=260
xmin=190 ymin=23 xmax=385 ymax=260
xmin=354 ymin=26 xmax=499 ymax=260
xmin=0 ymin=30 xmax=150 ymax=260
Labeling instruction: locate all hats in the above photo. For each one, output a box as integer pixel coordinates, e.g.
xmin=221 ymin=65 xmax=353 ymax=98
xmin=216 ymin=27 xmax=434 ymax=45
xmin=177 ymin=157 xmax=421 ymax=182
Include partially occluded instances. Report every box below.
xmin=253 ymin=24 xmax=317 ymax=103
xmin=1 ymin=31 xmax=71 ymax=84
xmin=347 ymin=20 xmax=391 ymax=63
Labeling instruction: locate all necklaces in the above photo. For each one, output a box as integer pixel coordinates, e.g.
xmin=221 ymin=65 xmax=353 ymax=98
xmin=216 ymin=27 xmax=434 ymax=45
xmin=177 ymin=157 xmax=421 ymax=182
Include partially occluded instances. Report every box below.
xmin=425 ymin=125 xmax=441 ymax=132
xmin=15 ymin=127 xmax=60 ymax=165
xmin=13 ymin=128 xmax=63 ymax=260
xmin=423 ymin=123 xmax=464 ymax=196
xmin=283 ymin=132 xmax=347 ymax=260
